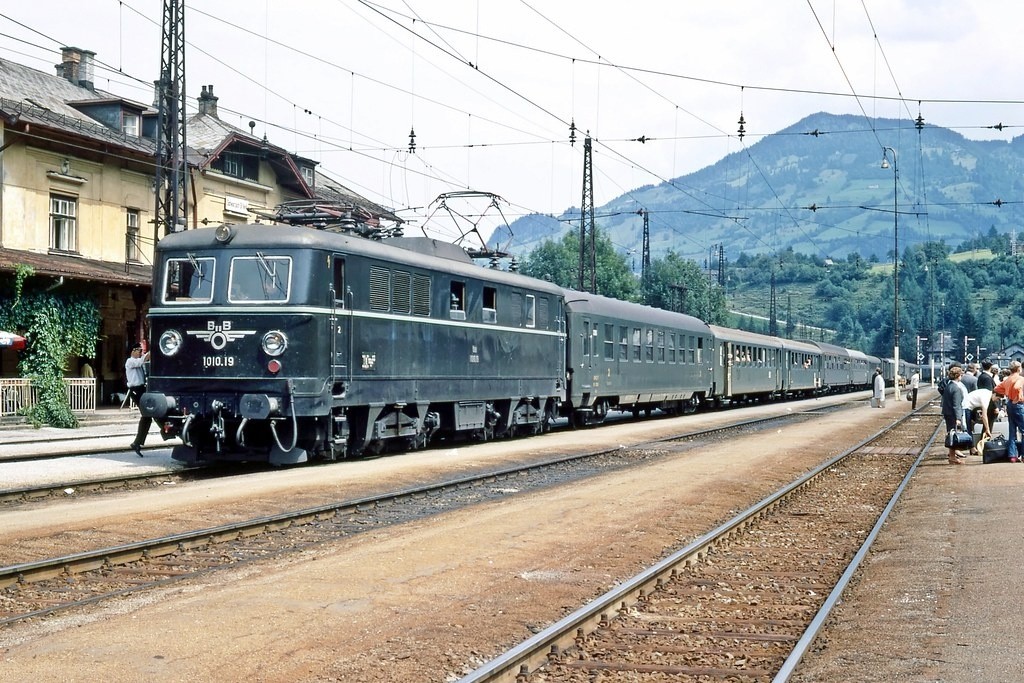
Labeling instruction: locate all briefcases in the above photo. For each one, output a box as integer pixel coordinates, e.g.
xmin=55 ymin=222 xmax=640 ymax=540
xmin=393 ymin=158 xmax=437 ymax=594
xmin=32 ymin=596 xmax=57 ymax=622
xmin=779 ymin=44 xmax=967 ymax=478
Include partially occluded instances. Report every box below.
xmin=871 ymin=397 xmax=885 ymax=408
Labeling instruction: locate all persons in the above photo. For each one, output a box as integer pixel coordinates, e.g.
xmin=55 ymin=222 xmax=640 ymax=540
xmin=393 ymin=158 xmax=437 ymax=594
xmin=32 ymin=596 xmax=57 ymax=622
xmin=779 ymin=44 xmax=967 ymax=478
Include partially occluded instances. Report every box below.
xmin=263 ymin=280 xmax=285 ymax=300
xmin=125 ymin=343 xmax=152 ymax=457
xmin=938 ymin=360 xmax=1024 ymax=464
xmin=910 ymin=370 xmax=920 ymax=410
xmin=804 ymin=357 xmax=811 ymax=368
xmin=81 ymin=356 xmax=94 ymax=408
xmin=735 ymin=349 xmax=751 ymax=361
xmin=872 ymin=367 xmax=885 ymax=407
xmin=893 ymin=374 xmax=901 ymax=386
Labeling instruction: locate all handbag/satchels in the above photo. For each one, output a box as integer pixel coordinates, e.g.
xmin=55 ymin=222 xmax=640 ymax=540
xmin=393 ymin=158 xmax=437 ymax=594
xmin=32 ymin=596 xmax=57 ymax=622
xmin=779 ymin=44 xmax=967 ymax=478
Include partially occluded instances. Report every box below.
xmin=907 ymin=389 xmax=913 ymax=401
xmin=997 ymin=409 xmax=1005 ymax=420
xmin=982 ymin=435 xmax=1022 ymax=464
xmin=945 ymin=424 xmax=974 ymax=451
xmin=977 ymin=432 xmax=991 ymax=456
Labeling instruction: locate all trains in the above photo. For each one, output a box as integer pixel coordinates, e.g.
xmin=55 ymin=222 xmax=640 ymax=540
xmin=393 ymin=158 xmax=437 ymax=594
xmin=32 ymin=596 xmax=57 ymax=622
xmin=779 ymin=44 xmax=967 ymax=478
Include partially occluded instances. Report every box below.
xmin=137 ymin=224 xmax=917 ymax=472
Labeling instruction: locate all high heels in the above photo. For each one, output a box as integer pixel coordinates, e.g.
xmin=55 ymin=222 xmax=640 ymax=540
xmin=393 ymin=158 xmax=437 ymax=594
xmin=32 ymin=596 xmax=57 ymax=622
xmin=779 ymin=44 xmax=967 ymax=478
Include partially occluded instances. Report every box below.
xmin=970 ymin=449 xmax=979 ymax=455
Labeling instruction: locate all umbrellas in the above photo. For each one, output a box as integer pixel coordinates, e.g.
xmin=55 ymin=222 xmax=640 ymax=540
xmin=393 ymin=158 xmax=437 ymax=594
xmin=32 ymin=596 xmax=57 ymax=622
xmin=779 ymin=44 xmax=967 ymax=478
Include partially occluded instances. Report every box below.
xmin=0 ymin=331 xmax=27 ymax=350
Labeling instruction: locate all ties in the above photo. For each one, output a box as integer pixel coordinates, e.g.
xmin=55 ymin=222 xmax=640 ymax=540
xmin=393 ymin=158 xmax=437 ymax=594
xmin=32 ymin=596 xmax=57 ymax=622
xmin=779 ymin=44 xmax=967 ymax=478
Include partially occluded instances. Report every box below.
xmin=141 ymin=366 xmax=146 ymax=383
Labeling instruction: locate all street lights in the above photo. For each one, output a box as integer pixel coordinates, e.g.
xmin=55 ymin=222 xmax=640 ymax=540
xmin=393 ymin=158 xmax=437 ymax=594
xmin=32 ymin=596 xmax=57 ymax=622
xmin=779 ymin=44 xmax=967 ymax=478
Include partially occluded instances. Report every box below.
xmin=878 ymin=146 xmax=901 ymax=402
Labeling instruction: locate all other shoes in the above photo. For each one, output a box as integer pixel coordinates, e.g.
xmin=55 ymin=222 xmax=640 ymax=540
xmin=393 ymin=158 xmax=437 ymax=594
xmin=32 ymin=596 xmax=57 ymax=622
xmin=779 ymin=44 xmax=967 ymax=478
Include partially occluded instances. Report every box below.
xmin=948 ymin=452 xmax=967 ymax=459
xmin=949 ymin=459 xmax=965 ymax=465
xmin=1010 ymin=457 xmax=1019 ymax=463
xmin=912 ymin=408 xmax=916 ymax=410
xmin=131 ymin=444 xmax=143 ymax=457
xmin=878 ymin=405 xmax=882 ymax=408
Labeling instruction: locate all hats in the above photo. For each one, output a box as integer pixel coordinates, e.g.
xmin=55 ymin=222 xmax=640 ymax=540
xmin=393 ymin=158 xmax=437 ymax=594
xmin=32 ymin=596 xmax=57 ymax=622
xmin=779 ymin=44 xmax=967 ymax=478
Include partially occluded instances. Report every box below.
xmin=129 ymin=343 xmax=145 ymax=351
